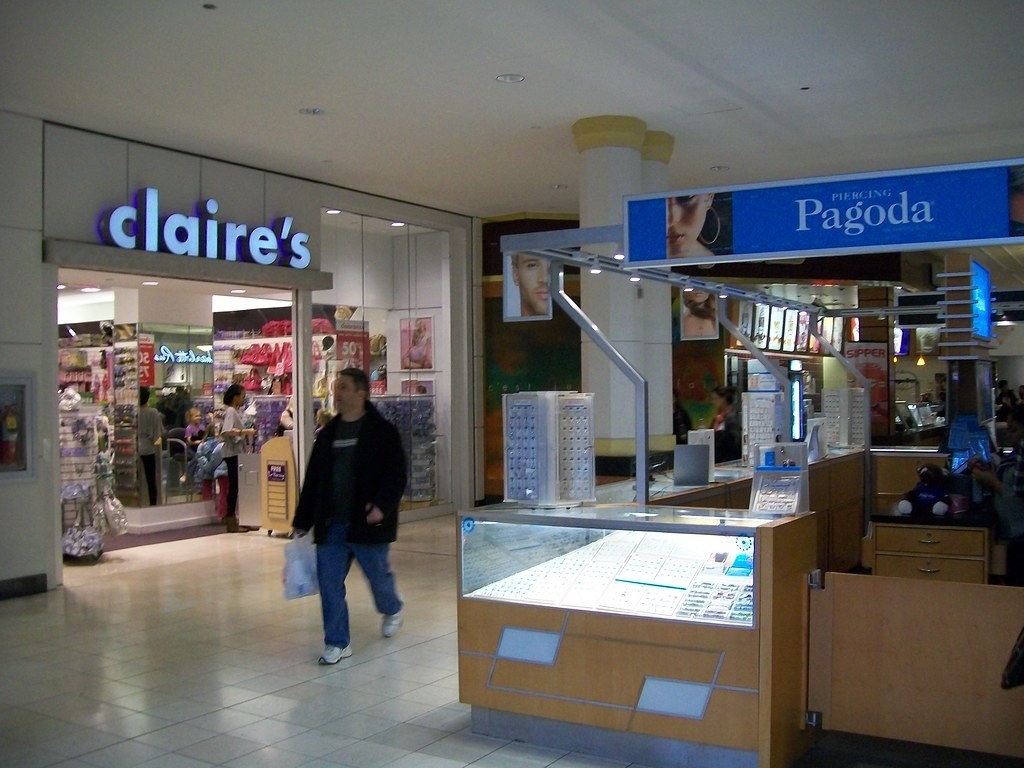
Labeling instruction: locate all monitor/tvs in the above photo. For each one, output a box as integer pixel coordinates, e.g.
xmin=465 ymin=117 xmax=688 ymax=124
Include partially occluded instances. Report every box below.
xmin=969 ymin=260 xmax=991 ymax=339
xmin=896 ymin=291 xmax=947 ymax=328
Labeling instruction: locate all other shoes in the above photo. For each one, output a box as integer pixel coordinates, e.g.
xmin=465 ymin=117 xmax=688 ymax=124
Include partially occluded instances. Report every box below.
xmin=111 ymin=346 xmax=138 ymax=388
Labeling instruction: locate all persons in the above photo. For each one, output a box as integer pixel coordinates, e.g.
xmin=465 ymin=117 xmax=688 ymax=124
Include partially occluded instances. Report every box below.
xmin=402 ymin=319 xmax=432 ymax=369
xmin=683 ymin=286 xmax=716 ymax=337
xmin=135 ymin=386 xmax=162 ymax=505
xmin=511 ymin=252 xmax=549 ymax=317
xmin=667 ymin=192 xmax=724 ymax=260
xmin=180 ymin=408 xmax=206 ymax=484
xmin=197 ymin=424 xmax=217 ymax=500
xmin=208 ymin=428 xmax=228 ymax=520
xmin=995 ymin=380 xmax=1024 ymax=424
xmin=672 ymin=386 xmax=695 ymax=444
xmin=314 ymin=408 xmax=334 ymax=438
xmin=221 ymin=385 xmax=255 ymax=532
xmin=274 ymin=396 xmax=292 ymax=439
xmin=292 ymin=368 xmax=409 ymax=664
xmin=706 ymin=386 xmax=742 ymax=464
xmin=967 ymin=404 xmax=1024 ymax=587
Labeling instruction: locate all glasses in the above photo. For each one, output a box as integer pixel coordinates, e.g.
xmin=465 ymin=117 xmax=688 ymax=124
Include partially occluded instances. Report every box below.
xmin=378 ymin=398 xmax=439 ymax=499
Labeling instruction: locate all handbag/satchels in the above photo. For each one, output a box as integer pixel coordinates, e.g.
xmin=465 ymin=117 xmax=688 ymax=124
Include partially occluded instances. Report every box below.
xmin=59 ymin=389 xmax=128 ymax=564
xmin=215 ymin=317 xmax=333 ymax=399
xmin=64 ymin=349 xmax=93 ymax=373
xmin=282 ymin=527 xmax=320 ymax=600
xmin=368 ymin=333 xmax=389 ymax=358
xmin=369 ymin=364 xmax=388 ymax=381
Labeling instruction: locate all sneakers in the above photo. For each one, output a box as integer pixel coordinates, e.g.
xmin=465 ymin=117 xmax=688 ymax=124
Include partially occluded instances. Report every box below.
xmin=382 ymin=593 xmax=405 ymax=638
xmin=316 ymin=645 xmax=353 ymax=664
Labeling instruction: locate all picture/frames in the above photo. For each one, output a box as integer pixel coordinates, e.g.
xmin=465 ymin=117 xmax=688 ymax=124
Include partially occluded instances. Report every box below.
xmin=399 ymin=315 xmax=435 ymax=369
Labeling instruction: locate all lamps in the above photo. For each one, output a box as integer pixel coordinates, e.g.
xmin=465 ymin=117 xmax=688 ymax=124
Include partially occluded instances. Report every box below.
xmin=916 ymin=355 xmax=926 ymax=366
xmin=878 ymin=313 xmax=885 ymax=320
xmin=590 ymin=264 xmax=602 ymax=275
xmin=636 ymin=286 xmax=645 ymax=300
xmin=629 ymin=272 xmax=641 ymax=281
xmin=613 ymin=247 xmax=625 ymax=260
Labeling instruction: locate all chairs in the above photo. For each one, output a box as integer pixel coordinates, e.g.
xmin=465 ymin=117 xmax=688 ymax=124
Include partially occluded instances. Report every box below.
xmin=164 ymin=428 xmax=197 ymax=505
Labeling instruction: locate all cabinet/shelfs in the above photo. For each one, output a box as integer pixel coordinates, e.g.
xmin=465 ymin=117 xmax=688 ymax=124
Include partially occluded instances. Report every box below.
xmin=873 ymin=523 xmax=988 ymax=584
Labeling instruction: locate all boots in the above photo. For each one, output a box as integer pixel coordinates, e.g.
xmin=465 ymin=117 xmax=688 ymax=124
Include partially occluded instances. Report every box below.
xmin=226 ymin=517 xmax=250 ymax=533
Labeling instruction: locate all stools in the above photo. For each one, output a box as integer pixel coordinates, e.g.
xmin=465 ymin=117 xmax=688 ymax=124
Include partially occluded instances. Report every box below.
xmin=61 ymin=494 xmax=94 ymax=535
